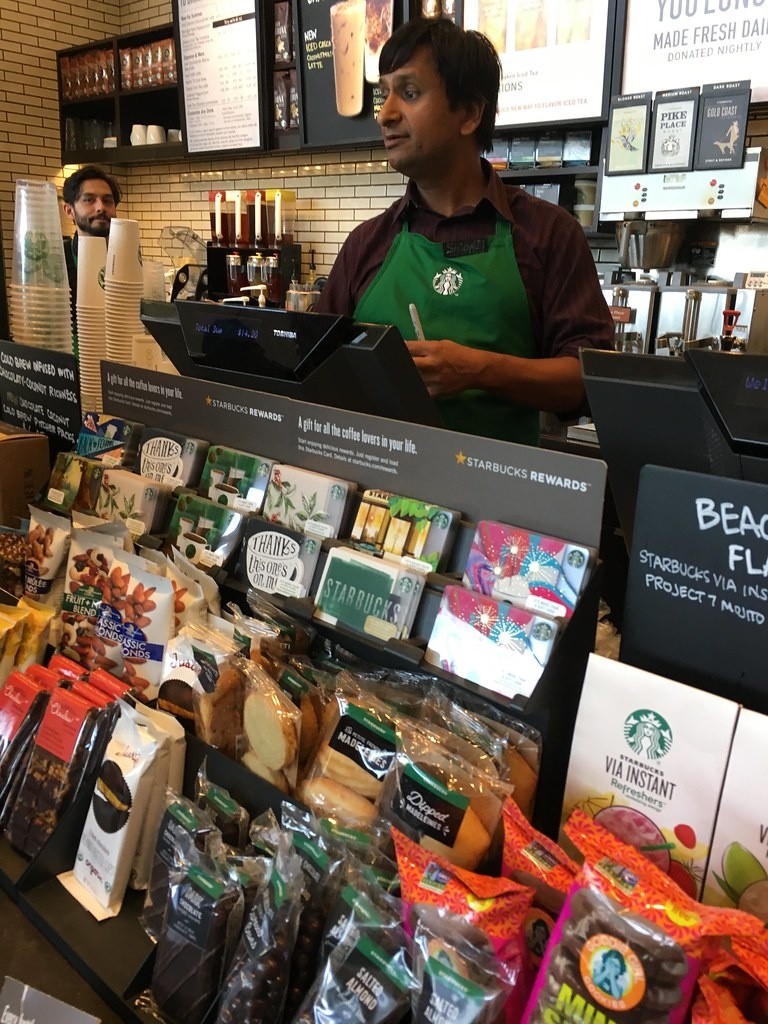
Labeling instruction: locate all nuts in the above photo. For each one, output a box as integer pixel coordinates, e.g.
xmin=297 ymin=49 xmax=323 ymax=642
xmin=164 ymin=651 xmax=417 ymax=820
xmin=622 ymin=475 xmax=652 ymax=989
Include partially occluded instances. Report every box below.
xmin=24 ymin=526 xmax=189 ymax=702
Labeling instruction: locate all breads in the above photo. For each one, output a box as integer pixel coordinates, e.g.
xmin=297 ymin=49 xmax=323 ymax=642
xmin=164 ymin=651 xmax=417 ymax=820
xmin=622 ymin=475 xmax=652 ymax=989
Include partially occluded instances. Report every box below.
xmin=192 ymin=649 xmax=534 ymax=874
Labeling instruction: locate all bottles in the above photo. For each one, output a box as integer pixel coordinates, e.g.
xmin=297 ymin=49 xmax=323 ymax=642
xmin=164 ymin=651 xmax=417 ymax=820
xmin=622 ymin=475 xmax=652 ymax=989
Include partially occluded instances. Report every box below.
xmin=65 ymin=118 xmax=115 ymax=152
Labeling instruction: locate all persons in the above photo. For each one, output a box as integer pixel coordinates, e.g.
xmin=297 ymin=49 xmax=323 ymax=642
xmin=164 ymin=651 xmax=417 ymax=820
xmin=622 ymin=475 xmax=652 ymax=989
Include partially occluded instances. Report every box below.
xmin=308 ymin=17 xmax=615 ymax=445
xmin=62 ymin=165 xmax=123 ymax=357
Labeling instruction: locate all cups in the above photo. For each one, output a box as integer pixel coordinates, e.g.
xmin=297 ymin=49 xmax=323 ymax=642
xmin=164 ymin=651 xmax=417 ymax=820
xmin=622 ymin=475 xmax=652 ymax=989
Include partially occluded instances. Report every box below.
xmin=130 ymin=124 xmax=182 ymax=146
xmin=7 ymin=180 xmax=166 ymax=426
xmin=329 ymin=0 xmax=365 ymax=117
xmin=362 ymin=0 xmax=392 ymax=82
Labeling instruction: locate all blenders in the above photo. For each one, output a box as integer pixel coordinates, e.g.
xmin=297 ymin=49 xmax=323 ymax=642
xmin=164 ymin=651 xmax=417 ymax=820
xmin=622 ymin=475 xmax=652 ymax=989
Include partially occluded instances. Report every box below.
xmin=208 ymin=188 xmax=295 ymax=310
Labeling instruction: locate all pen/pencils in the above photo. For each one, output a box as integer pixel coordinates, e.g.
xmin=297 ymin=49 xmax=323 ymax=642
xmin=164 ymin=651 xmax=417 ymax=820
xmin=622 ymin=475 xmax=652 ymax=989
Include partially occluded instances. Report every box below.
xmin=409 ymin=303 xmax=425 ymax=341
